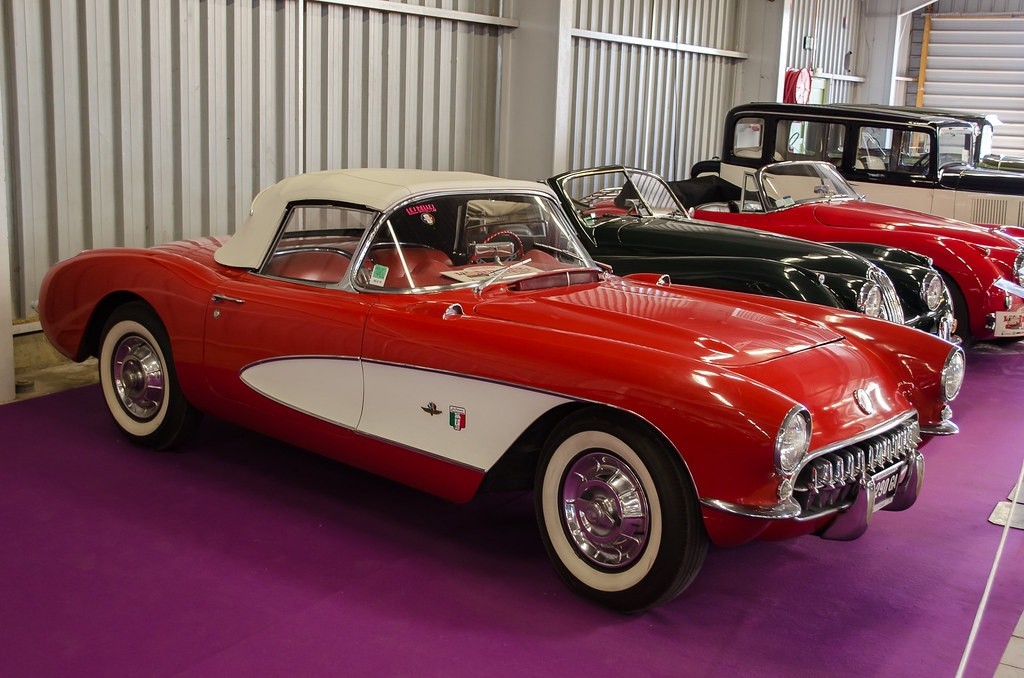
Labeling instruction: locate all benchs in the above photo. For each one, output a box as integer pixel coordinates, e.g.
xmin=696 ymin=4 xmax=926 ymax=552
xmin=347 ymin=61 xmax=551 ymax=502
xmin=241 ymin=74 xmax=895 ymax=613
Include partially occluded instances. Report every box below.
xmin=616 ymin=172 xmax=753 ymax=217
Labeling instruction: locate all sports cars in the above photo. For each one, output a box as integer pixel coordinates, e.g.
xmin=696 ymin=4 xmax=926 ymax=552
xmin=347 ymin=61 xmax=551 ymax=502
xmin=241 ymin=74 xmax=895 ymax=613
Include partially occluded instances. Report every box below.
xmin=34 ymin=161 xmax=966 ymax=616
xmin=466 ymin=164 xmax=962 ymax=355
xmin=579 ymin=160 xmax=1023 ymax=344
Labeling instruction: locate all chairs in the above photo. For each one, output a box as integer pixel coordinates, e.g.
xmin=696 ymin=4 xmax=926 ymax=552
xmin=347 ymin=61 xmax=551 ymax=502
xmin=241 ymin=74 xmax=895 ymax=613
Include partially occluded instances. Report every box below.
xmin=280 ymin=241 xmax=455 ymax=289
xmin=737 ymin=146 xmax=888 ymax=171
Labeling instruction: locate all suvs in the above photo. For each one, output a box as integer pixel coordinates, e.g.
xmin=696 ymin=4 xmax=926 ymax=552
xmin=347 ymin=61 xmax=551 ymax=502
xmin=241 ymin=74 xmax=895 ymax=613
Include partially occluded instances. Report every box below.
xmin=806 ymin=100 xmax=1024 ymax=172
xmin=690 ymin=103 xmax=1024 ymax=227
